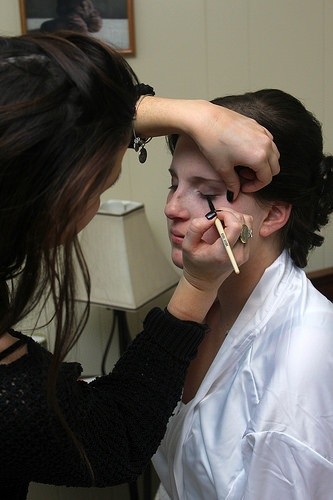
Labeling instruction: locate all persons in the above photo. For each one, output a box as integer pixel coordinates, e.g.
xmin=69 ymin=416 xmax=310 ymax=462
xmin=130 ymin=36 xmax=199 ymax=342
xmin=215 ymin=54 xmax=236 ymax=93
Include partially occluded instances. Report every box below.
xmin=0 ymin=6 xmax=283 ymax=499
xmin=152 ymin=85 xmax=333 ymax=500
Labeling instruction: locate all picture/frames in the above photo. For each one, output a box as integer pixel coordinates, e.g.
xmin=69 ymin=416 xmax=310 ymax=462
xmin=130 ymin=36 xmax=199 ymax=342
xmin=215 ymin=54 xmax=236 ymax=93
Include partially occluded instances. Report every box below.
xmin=18 ymin=0 xmax=136 ymax=56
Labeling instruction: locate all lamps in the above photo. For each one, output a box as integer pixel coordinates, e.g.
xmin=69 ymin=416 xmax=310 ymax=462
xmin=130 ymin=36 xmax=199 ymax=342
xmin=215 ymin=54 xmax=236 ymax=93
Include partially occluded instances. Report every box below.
xmin=46 ymin=197 xmax=180 ymax=377
